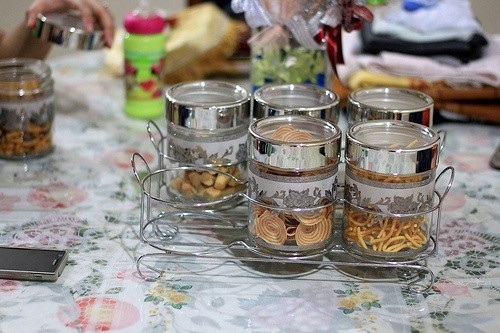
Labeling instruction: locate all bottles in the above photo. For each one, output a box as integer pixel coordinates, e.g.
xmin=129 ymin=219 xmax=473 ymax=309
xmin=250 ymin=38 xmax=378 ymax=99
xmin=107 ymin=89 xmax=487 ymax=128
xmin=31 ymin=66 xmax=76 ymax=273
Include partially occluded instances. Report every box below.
xmin=122 ymin=0 xmax=164 ymax=121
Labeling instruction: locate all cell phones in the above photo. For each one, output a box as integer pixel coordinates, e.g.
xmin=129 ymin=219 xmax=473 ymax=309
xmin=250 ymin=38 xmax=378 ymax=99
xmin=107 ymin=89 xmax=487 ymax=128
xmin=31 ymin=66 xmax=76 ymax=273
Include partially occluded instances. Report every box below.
xmin=0 ymin=245 xmax=68 ymax=281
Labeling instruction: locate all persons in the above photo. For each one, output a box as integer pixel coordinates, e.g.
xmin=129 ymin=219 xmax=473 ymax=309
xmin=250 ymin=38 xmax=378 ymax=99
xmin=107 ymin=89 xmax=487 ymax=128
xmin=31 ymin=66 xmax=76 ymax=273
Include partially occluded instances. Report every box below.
xmin=0 ymin=0 xmax=114 ymax=60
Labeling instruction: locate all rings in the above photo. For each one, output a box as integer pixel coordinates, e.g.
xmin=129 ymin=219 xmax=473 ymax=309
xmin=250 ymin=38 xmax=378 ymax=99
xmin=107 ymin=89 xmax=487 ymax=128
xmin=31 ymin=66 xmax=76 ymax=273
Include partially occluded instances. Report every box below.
xmin=103 ymin=3 xmax=109 ymax=8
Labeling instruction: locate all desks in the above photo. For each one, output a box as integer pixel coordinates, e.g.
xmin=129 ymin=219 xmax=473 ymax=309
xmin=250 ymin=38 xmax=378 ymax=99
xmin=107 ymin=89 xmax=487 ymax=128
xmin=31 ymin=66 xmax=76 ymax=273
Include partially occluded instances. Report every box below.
xmin=0 ymin=52 xmax=500 ymax=333
